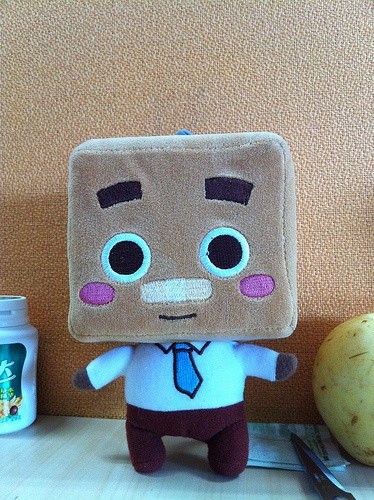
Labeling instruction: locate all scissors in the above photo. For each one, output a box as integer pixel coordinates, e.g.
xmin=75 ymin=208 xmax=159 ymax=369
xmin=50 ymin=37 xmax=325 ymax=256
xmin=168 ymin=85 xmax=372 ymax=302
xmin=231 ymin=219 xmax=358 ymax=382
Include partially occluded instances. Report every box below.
xmin=289 ymin=431 xmax=355 ymax=500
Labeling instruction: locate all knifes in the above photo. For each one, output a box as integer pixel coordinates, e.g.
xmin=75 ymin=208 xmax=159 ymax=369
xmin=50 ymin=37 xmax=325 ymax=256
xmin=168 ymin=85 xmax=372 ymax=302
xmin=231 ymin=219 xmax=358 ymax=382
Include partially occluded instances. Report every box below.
xmin=291 ymin=433 xmax=356 ymax=500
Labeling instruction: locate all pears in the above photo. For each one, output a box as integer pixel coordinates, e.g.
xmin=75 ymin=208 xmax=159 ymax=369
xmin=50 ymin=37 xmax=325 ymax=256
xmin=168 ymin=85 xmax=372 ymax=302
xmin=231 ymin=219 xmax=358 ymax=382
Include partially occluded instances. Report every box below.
xmin=312 ymin=313 xmax=374 ymax=468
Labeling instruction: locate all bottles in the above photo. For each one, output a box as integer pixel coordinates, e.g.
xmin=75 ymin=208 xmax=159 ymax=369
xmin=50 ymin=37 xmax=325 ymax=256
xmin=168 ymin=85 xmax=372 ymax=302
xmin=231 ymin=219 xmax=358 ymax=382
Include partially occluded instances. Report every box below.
xmin=1 ymin=295 xmax=37 ymax=435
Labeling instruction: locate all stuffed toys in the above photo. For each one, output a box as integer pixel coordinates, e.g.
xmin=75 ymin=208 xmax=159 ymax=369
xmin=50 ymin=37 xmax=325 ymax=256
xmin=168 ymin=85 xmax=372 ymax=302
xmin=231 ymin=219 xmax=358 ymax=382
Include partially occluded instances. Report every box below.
xmin=70 ymin=132 xmax=298 ymax=477
